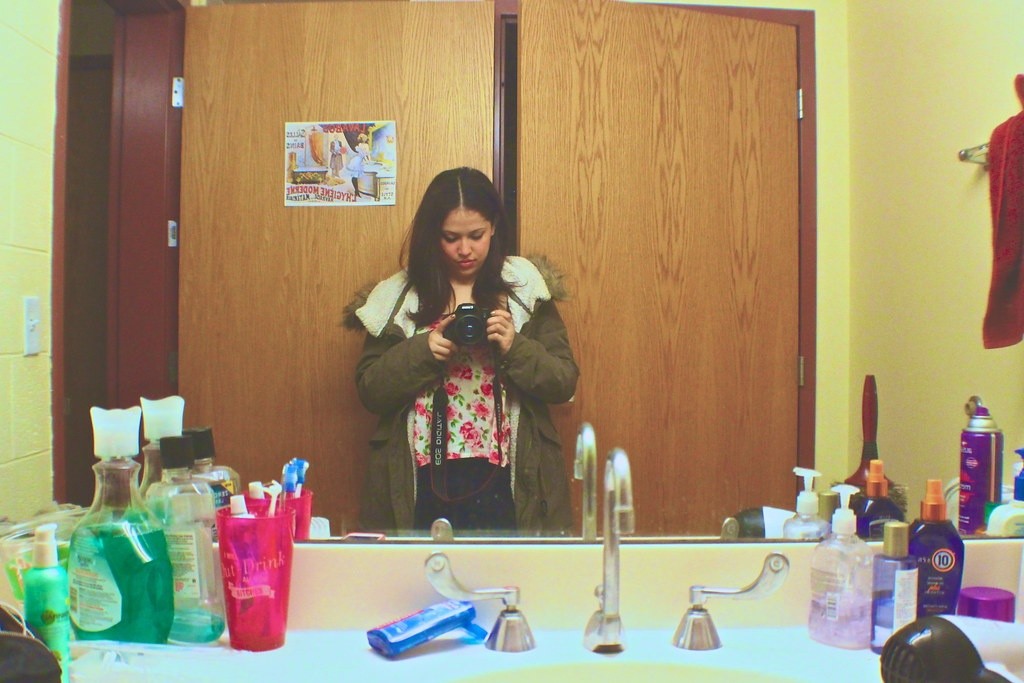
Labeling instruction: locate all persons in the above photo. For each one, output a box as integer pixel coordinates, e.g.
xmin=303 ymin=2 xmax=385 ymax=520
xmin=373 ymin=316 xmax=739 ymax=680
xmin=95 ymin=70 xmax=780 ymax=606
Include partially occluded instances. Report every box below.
xmin=337 ymin=167 xmax=579 ymax=538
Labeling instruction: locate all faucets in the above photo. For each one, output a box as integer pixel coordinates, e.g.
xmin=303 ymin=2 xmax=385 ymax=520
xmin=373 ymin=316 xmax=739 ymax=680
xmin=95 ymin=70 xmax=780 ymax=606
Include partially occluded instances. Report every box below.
xmin=574 ymin=421 xmax=598 ymax=544
xmin=583 ymin=446 xmax=636 ymax=656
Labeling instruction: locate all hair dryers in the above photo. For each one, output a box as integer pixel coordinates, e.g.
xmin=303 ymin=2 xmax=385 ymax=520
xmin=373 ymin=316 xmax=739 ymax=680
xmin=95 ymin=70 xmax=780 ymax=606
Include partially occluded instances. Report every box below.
xmin=720 ymin=506 xmax=797 ymax=540
xmin=879 ymin=614 xmax=1024 ymax=683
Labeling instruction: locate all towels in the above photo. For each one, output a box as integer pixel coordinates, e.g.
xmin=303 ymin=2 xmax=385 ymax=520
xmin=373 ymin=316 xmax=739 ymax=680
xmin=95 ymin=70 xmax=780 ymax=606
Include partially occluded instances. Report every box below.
xmin=980 ymin=74 xmax=1024 ymax=351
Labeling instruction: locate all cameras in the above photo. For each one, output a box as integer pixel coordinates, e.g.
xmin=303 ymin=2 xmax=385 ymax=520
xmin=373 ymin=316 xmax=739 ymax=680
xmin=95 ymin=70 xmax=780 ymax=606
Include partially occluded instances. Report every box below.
xmin=442 ymin=302 xmax=498 ymax=346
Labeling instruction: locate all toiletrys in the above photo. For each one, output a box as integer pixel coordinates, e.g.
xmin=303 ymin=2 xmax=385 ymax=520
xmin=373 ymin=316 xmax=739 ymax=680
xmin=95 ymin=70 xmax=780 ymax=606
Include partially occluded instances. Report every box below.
xmin=21 ymin=406 xmax=226 ymax=683
xmin=782 ymin=406 xmax=1024 ymax=538
xmin=807 ymin=477 xmax=1017 ymax=655
xmin=225 ymin=494 xmax=255 ymax=519
xmin=366 ymin=599 xmax=488 ymax=658
xmin=134 ymin=394 xmax=241 ymax=542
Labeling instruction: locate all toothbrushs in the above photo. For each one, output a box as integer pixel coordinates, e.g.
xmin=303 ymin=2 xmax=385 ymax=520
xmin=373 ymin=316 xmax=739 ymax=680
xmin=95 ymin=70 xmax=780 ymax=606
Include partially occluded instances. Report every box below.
xmin=280 ymin=457 xmax=310 ymax=500
xmin=261 ymin=479 xmax=283 ymax=518
